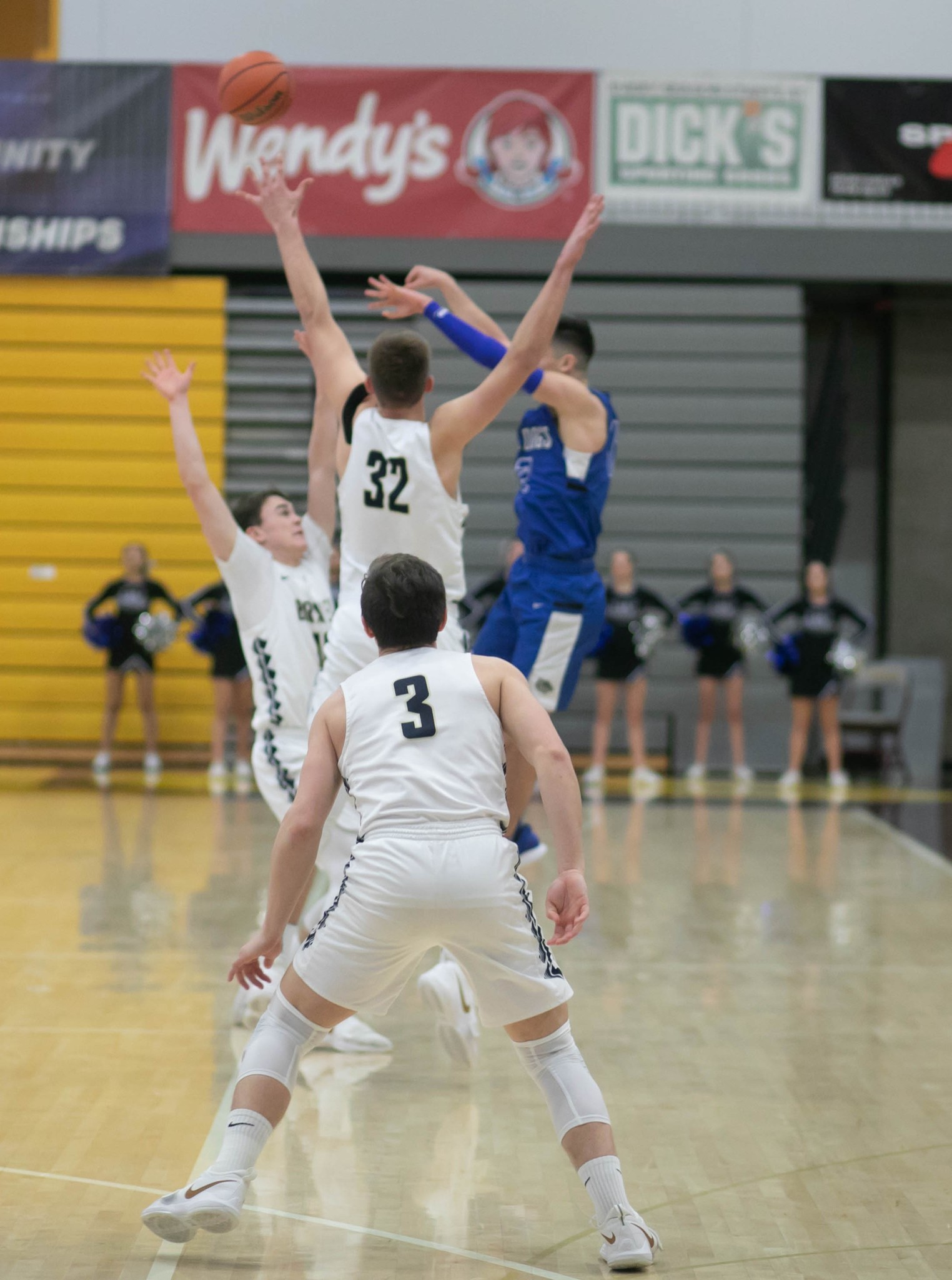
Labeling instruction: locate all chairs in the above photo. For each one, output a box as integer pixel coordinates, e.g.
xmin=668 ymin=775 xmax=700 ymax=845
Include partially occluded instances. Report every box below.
xmin=810 ymin=671 xmax=918 ymax=788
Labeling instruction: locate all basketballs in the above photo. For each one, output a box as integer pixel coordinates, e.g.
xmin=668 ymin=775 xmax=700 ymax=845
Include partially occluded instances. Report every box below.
xmin=218 ymin=50 xmax=293 ymax=126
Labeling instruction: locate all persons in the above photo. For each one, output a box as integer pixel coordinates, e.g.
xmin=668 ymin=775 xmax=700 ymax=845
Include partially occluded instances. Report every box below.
xmin=231 ymin=151 xmax=606 ymax=1068
xmin=142 ymin=555 xmax=659 ymax=1271
xmin=84 ymin=542 xmax=853 ymax=790
xmin=363 ymin=265 xmax=619 ymax=867
xmin=147 ymin=328 xmax=399 ymax=1056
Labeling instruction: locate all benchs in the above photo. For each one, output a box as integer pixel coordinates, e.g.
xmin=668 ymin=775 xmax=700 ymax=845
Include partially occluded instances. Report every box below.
xmin=562 ymin=702 xmax=679 ymax=775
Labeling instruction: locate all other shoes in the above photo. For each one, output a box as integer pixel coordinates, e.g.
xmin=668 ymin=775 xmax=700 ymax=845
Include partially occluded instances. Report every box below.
xmin=687 ymin=767 xmax=703 ymax=779
xmin=143 ymin=754 xmax=162 ymax=770
xmin=95 ymin=754 xmax=109 ymax=767
xmin=631 ymin=767 xmax=660 ymax=788
xmin=826 ymin=770 xmax=849 ymax=787
xmin=732 ymin=767 xmax=753 ymax=781
xmin=208 ymin=763 xmax=224 ymax=777
xmin=234 ymin=761 xmax=250 ymax=776
xmin=779 ymin=772 xmax=800 ymax=785
xmin=580 ymin=769 xmax=603 ymax=781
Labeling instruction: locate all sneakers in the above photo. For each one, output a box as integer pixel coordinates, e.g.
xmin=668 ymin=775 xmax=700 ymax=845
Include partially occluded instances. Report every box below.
xmin=297 ymin=1051 xmax=392 ymax=1085
xmin=512 ymin=824 xmax=547 ymax=863
xmin=241 ymin=963 xmax=287 ymax=1000
xmin=318 ymin=1016 xmax=394 ymax=1052
xmin=417 ymin=1108 xmax=478 ymax=1217
xmin=418 ymin=960 xmax=480 ymax=1072
xmin=598 ymin=1204 xmax=663 ymax=1269
xmin=140 ymin=1167 xmax=257 ymax=1243
